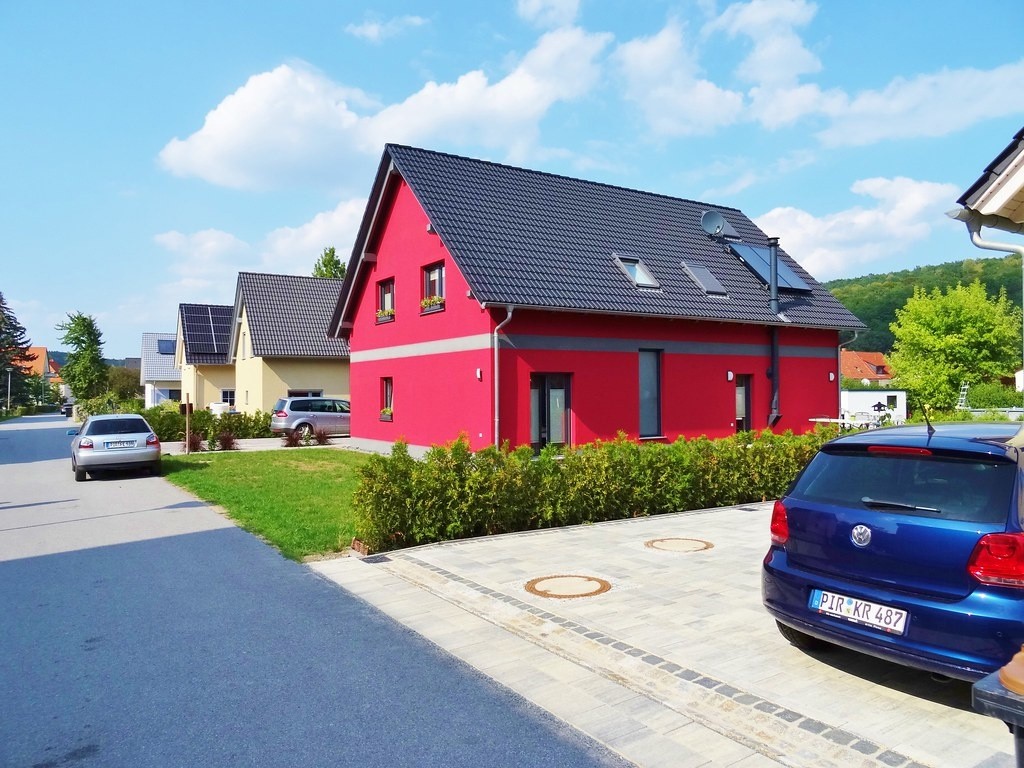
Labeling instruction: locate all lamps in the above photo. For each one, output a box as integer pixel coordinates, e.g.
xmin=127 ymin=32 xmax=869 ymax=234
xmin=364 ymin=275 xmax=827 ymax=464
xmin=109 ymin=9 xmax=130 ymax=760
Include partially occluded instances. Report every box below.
xmin=872 ymin=401 xmax=888 ymax=428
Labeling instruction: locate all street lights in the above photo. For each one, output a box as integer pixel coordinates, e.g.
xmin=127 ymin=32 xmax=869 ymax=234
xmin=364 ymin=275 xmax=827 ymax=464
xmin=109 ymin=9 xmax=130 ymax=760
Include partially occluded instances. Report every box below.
xmin=5 ymin=368 xmax=14 ymax=410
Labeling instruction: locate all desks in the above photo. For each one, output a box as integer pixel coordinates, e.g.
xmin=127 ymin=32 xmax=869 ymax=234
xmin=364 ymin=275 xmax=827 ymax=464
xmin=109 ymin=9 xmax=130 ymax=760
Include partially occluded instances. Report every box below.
xmin=809 ymin=418 xmax=875 ymax=434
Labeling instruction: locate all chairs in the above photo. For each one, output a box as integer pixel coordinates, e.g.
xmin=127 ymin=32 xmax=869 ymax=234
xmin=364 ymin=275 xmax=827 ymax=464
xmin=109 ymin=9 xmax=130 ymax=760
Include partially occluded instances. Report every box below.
xmin=320 ymin=405 xmax=328 ymax=411
xmin=855 ymin=412 xmax=869 ymax=420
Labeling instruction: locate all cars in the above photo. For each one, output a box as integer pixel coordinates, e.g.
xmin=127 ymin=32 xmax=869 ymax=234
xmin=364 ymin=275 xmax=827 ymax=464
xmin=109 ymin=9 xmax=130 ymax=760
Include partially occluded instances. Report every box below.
xmin=61 ymin=403 xmax=73 ymax=415
xmin=269 ymin=396 xmax=350 ymax=440
xmin=758 ymin=421 xmax=1024 ymax=685
xmin=66 ymin=414 xmax=162 ymax=483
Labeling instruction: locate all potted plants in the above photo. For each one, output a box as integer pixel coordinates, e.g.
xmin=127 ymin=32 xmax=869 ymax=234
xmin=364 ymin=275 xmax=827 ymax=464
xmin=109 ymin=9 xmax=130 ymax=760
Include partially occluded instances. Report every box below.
xmin=419 ymin=296 xmax=445 ymax=313
xmin=379 ymin=407 xmax=392 ymax=420
xmin=375 ymin=309 xmax=395 ymax=323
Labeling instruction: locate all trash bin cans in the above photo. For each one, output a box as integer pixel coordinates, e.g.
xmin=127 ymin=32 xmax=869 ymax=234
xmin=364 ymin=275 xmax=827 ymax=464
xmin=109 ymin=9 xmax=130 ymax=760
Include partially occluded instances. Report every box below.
xmin=66 ymin=408 xmax=72 ymax=417
